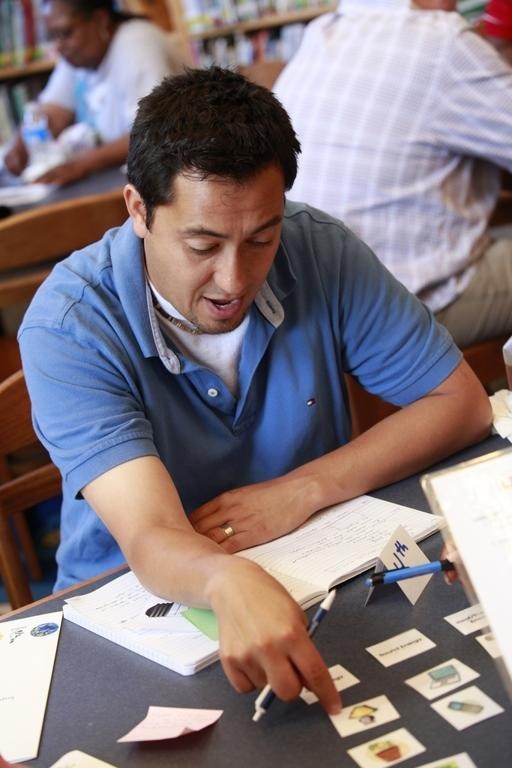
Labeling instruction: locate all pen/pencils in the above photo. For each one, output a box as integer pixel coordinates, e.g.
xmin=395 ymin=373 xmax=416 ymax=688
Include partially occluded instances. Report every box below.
xmin=366 ymin=558 xmax=452 ymax=588
xmin=251 ymin=586 xmax=340 ymax=725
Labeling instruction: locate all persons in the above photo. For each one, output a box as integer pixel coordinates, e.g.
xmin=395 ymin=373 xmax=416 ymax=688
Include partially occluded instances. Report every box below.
xmin=15 ymin=61 xmax=495 ymax=716
xmin=436 ymin=542 xmax=460 ymax=590
xmin=4 ymin=2 xmax=188 ymax=195
xmin=266 ymin=2 xmax=510 ymax=346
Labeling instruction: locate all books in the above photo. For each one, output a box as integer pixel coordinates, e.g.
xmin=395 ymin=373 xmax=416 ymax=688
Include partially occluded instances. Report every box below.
xmin=63 ymin=493 xmax=446 ymax=677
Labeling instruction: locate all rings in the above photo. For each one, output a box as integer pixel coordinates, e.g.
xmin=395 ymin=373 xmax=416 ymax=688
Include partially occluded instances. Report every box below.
xmin=217 ymin=523 xmax=238 ymax=537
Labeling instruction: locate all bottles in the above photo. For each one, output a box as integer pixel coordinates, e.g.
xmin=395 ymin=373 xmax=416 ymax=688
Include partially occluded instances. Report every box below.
xmin=19 ymin=101 xmax=55 ymax=164
